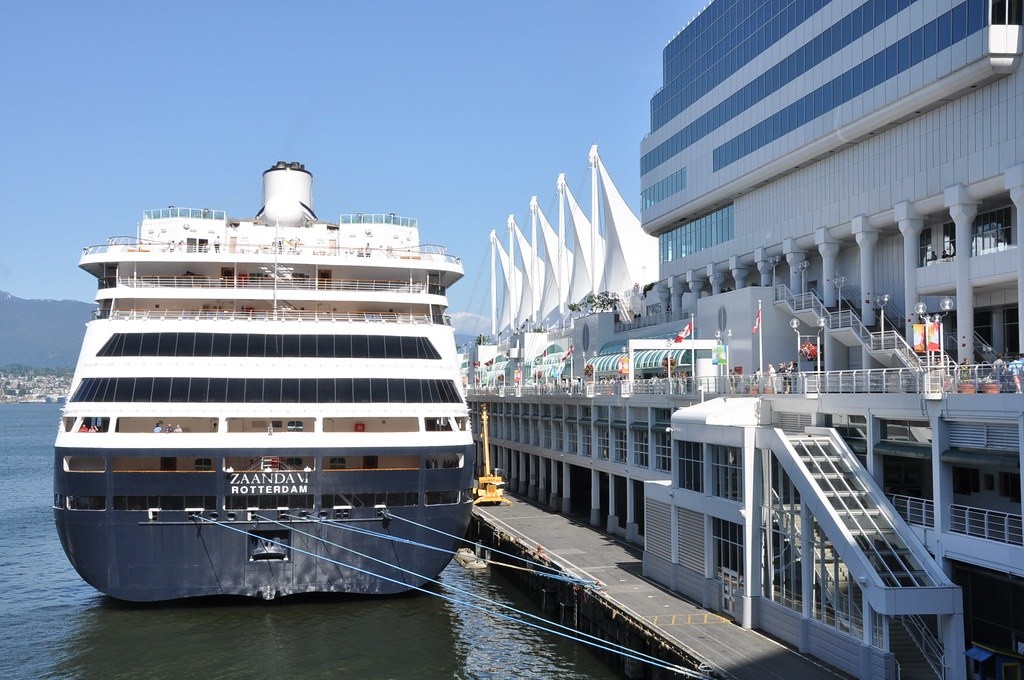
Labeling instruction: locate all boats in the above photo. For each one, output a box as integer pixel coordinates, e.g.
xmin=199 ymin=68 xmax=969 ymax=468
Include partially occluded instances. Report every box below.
xmin=51 ymin=161 xmax=476 ymax=600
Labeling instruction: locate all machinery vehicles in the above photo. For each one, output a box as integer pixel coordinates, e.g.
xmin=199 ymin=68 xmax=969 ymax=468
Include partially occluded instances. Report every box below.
xmin=473 ymin=402 xmax=511 ymax=506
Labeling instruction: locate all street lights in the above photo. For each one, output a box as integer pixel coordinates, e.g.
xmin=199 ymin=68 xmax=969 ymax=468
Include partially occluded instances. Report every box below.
xmin=833 ymin=276 xmax=848 ymax=328
xmin=796 ymin=260 xmax=810 ymax=310
xmin=714 ymin=328 xmax=734 ymax=375
xmin=874 ymin=293 xmax=891 ymax=350
xmin=790 ymin=317 xmax=827 ymax=370
xmin=767 ymin=255 xmax=781 ymax=300
xmin=583 ymin=350 xmax=597 ymax=381
xmin=621 ymin=345 xmax=633 ymax=380
xmin=665 ymin=336 xmax=674 ymax=378
xmin=914 ymin=298 xmax=953 ymax=374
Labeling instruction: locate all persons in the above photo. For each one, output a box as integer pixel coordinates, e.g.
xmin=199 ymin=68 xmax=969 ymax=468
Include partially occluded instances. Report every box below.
xmin=153 ymin=422 xmax=162 ymax=432
xmin=942 ymin=250 xmax=949 ymax=258
xmin=1007 ymin=355 xmax=1023 ymax=393
xmin=959 ymin=358 xmax=972 ymax=378
xmin=166 ymin=424 xmax=174 ymax=432
xmin=214 ymin=236 xmax=221 ymax=252
xmin=993 ymin=352 xmax=1007 ymax=393
xmin=364 ymin=242 xmax=372 ymax=257
xmin=935 ymin=357 xmax=945 ymax=371
xmin=80 ymin=424 xmax=89 ymax=433
xmin=174 ymin=425 xmax=183 ymax=432
xmin=1020 ymin=353 xmax=1024 ymax=375
xmin=88 ymin=425 xmax=98 ymax=432
xmin=927 ymin=251 xmax=938 ymax=261
xmin=729 ymin=361 xmax=794 ymax=394
xmin=601 ymin=373 xmax=685 ymax=394
xmin=427 ymin=457 xmax=461 ymax=468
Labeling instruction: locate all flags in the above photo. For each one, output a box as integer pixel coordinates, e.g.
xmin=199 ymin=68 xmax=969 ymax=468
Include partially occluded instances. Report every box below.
xmin=674 ymin=321 xmax=692 ymax=342
xmin=485 ymin=359 xmax=494 ymax=366
xmin=473 ymin=361 xmax=481 ymax=368
xmin=753 ymin=309 xmax=761 ymax=334
xmin=563 ymin=343 xmax=574 ymax=361
